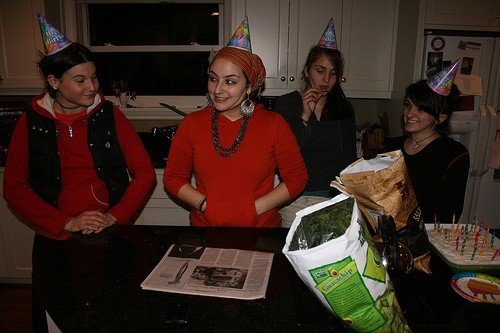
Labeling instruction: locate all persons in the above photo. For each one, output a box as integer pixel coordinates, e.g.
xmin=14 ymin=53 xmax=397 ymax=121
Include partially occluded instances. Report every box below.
xmin=362 ymin=57 xmax=470 ymax=224
xmin=2 ymin=14 xmax=156 ymax=333
xmin=270 ymin=17 xmax=356 ymax=227
xmin=162 ymin=15 xmax=308 ymax=228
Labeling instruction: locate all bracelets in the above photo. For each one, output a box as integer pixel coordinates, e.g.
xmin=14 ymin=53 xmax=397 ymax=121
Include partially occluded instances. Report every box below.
xmin=302 ymin=118 xmax=308 ymax=124
xmin=198 ymin=197 xmax=205 ymax=210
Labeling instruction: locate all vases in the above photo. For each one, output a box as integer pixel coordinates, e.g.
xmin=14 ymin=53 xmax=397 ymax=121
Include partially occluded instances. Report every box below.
xmin=119 ymin=92 xmax=128 ymax=108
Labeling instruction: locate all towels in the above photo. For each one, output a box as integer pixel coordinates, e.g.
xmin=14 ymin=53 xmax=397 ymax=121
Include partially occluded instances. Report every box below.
xmin=454 ymin=75 xmax=483 ymax=98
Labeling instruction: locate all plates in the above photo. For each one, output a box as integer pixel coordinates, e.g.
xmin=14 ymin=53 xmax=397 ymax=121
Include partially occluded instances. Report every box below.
xmin=450 ymin=272 xmax=500 ymax=304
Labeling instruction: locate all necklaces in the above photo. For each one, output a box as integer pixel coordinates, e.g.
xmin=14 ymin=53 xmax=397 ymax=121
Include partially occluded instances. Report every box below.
xmin=56 ymin=98 xmax=84 ymax=109
xmin=410 ymin=134 xmax=434 ymax=147
xmin=212 ymin=109 xmax=248 ymax=157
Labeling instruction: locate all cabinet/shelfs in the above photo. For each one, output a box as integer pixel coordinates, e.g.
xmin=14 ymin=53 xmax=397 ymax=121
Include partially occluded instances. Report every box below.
xmin=229 ymin=1 xmax=398 ymax=99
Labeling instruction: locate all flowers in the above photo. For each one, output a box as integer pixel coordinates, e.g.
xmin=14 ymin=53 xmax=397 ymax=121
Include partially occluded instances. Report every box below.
xmin=112 ymin=80 xmax=137 ymax=100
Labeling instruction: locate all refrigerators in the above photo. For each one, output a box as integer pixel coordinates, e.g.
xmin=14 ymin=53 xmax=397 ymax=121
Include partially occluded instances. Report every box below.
xmin=421 ymin=34 xmax=500 ymax=230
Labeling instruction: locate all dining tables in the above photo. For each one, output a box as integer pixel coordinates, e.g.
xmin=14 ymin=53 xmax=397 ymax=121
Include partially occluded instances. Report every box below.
xmin=35 ymin=224 xmax=500 ymax=333
